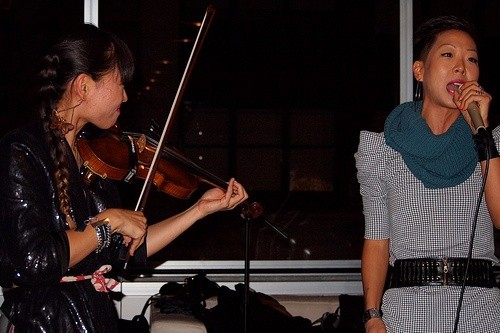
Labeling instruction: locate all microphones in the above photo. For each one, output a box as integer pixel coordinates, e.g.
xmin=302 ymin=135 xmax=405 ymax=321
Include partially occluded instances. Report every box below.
xmin=457 ymin=84 xmax=489 ymax=146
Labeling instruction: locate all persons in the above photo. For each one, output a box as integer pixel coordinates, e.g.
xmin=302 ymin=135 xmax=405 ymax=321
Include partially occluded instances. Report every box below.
xmin=0 ymin=23 xmax=249 ymax=333
xmin=352 ymin=15 xmax=500 ymax=333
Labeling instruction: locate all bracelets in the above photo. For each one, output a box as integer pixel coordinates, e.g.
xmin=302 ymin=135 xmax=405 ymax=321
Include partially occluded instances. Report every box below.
xmin=362 ymin=308 xmax=383 ymax=323
xmin=83 ymin=215 xmax=112 ymax=254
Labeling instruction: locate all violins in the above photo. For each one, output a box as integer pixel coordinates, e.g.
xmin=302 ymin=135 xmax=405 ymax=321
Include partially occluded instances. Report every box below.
xmin=75 ymin=119 xmax=263 ymax=223
